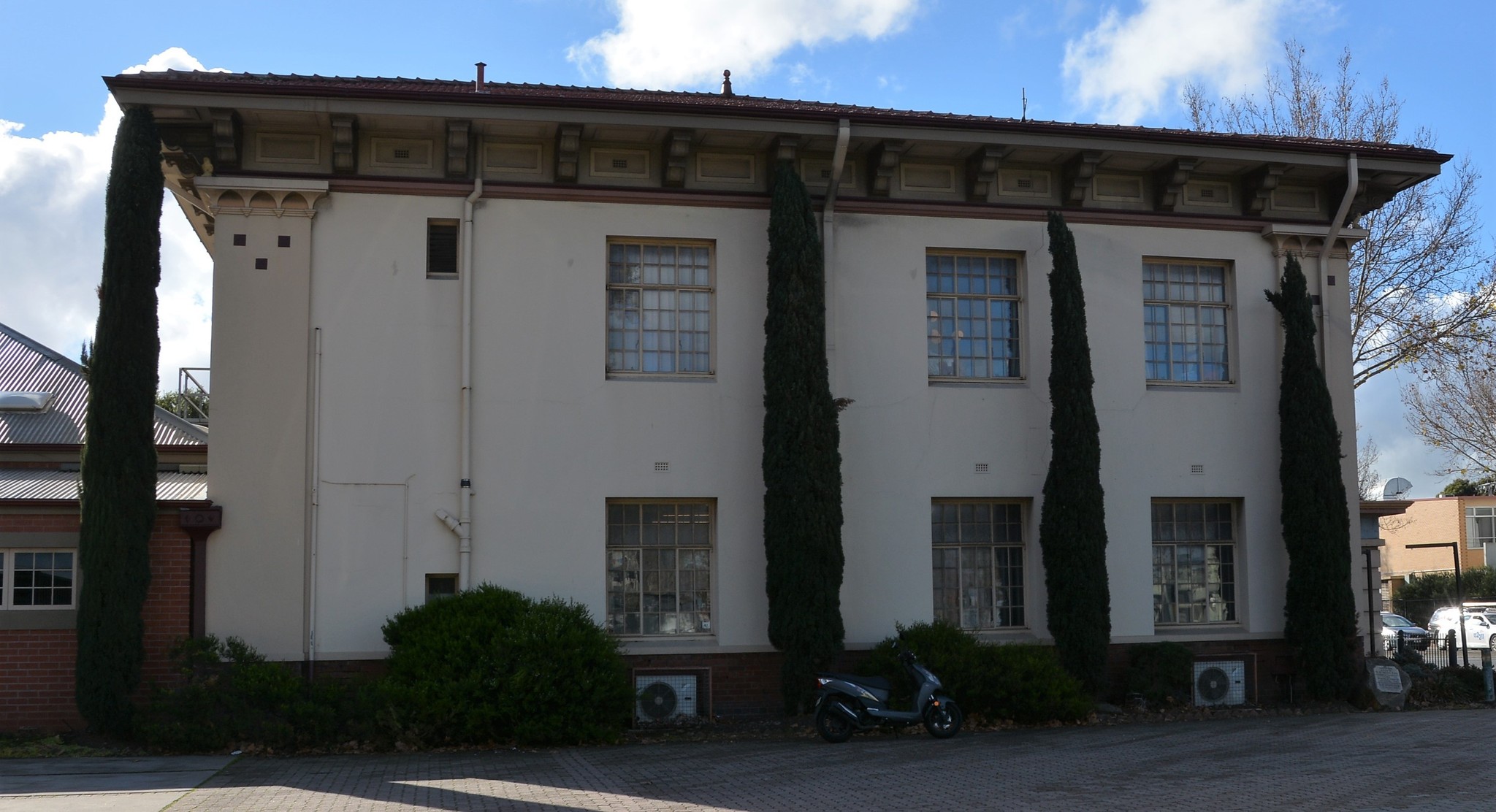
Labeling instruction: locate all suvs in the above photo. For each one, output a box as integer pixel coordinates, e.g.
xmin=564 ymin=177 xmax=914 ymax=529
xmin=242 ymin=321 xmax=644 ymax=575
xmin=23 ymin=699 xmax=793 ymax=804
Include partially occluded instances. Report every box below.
xmin=1428 ymin=606 xmax=1490 ymax=647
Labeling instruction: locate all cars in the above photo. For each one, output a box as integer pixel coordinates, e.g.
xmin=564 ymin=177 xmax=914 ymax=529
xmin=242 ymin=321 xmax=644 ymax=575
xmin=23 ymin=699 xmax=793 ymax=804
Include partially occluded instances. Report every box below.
xmin=1437 ymin=612 xmax=1496 ymax=652
xmin=1380 ymin=610 xmax=1431 ymax=652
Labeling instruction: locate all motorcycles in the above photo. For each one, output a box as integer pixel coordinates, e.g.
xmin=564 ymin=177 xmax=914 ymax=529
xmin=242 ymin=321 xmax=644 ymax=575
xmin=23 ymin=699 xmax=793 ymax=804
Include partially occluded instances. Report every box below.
xmin=811 ymin=647 xmax=963 ymax=744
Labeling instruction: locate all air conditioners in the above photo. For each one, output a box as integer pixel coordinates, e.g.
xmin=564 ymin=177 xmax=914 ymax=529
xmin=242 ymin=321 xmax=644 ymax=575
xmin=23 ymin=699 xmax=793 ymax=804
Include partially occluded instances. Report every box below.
xmin=635 ymin=675 xmax=697 ymax=728
xmin=1193 ymin=658 xmax=1246 ymax=707
